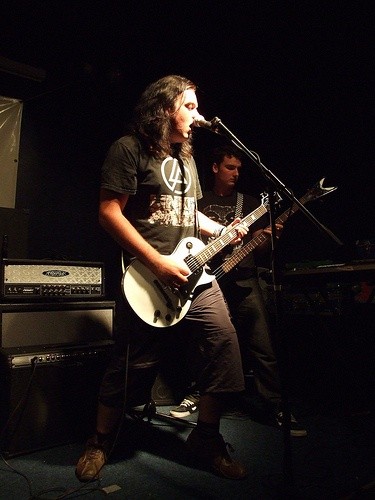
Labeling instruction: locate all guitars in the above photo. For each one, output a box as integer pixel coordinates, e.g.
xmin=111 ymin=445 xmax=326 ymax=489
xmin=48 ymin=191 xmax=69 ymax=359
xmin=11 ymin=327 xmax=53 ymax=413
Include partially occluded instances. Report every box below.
xmin=119 ymin=190 xmax=284 ymax=328
xmin=212 ymin=178 xmax=340 ymax=282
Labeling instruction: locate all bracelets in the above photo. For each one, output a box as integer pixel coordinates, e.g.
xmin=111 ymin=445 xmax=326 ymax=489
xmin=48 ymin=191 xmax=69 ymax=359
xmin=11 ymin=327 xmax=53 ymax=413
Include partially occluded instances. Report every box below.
xmin=212 ymin=224 xmax=227 ymax=240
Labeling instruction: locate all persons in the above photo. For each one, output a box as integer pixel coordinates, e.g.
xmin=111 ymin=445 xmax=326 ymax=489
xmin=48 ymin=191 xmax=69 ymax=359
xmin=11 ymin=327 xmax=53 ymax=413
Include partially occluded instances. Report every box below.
xmin=76 ymin=75 xmax=250 ymax=480
xmin=169 ymin=144 xmax=309 ymax=438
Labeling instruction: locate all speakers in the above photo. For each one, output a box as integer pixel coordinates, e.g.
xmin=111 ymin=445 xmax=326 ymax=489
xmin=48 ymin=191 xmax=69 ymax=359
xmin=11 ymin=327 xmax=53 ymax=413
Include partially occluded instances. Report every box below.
xmin=0 ymin=299 xmax=116 ymax=459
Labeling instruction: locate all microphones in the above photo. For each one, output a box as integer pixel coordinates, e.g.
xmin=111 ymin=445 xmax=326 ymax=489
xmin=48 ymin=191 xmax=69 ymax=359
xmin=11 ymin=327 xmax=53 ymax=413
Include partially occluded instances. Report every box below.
xmin=194 ymin=116 xmax=226 ymax=136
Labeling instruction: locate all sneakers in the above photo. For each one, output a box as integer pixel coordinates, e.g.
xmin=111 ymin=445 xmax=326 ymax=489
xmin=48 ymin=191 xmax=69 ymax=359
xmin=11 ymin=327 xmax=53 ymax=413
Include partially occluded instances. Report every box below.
xmin=170 ymin=391 xmax=200 ymax=417
xmin=76 ymin=435 xmax=115 ymax=482
xmin=197 ymin=442 xmax=249 ymax=480
xmin=272 ymin=412 xmax=306 ymax=436
